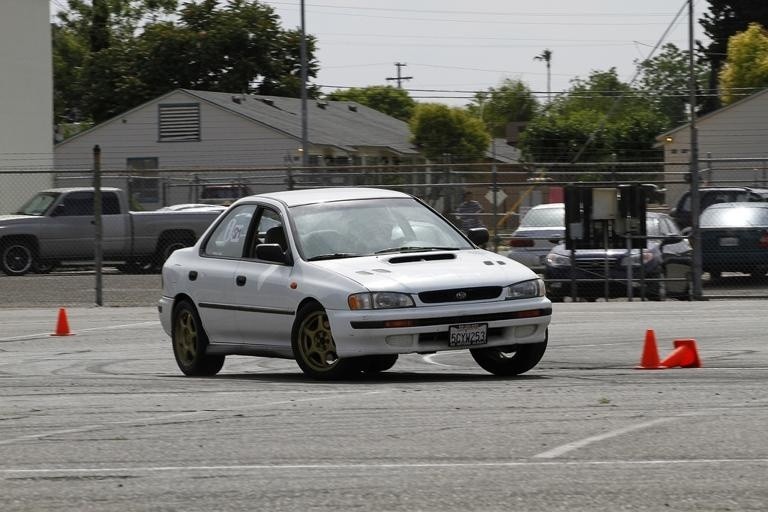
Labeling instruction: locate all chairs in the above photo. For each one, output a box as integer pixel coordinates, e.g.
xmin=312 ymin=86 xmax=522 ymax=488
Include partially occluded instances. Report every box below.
xmin=224 ymin=224 xmax=392 ymax=264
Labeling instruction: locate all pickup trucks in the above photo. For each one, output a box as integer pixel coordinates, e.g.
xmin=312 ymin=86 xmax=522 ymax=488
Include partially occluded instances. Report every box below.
xmin=0 ymin=186 xmax=222 ymax=276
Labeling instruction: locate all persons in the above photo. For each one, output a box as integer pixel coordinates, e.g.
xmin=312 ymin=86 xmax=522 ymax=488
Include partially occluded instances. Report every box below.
xmin=454 ymin=192 xmax=485 ymax=237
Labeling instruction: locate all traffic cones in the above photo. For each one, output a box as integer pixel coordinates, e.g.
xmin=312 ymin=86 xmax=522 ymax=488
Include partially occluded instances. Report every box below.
xmin=50 ymin=308 xmax=75 ymax=336
xmin=657 ymin=339 xmax=703 ymax=368
xmin=633 ymin=329 xmax=667 ymax=369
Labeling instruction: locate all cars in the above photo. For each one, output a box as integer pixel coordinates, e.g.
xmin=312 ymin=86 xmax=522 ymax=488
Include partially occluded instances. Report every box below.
xmin=159 ymin=186 xmax=552 ymax=382
xmin=699 ymin=202 xmax=768 ymax=279
xmin=545 ymin=212 xmax=694 ymax=302
xmin=510 ymin=202 xmax=566 ymax=274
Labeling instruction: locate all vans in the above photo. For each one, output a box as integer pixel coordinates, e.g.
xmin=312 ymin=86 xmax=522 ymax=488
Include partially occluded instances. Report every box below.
xmin=199 ymin=184 xmax=252 ymax=206
xmin=675 ymin=187 xmax=768 ymax=230
